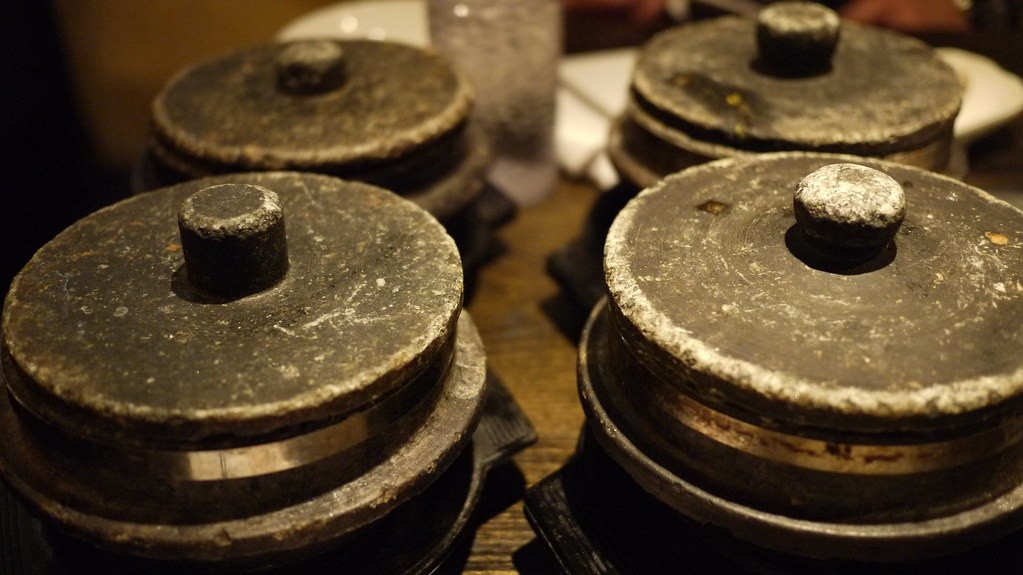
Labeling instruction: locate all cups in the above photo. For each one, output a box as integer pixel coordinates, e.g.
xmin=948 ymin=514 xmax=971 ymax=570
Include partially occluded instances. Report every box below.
xmin=428 ymin=0 xmax=559 ymax=203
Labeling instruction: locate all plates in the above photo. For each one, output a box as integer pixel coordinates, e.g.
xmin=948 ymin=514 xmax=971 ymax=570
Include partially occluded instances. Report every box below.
xmin=275 ymin=0 xmax=432 ymax=51
xmin=554 ymin=48 xmax=1023 ymax=140
